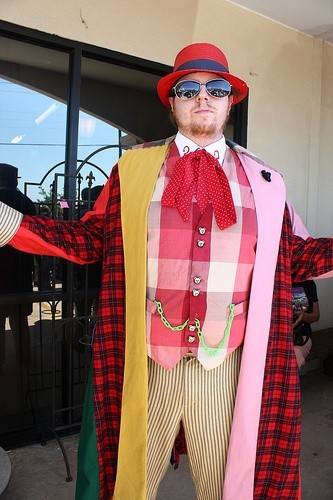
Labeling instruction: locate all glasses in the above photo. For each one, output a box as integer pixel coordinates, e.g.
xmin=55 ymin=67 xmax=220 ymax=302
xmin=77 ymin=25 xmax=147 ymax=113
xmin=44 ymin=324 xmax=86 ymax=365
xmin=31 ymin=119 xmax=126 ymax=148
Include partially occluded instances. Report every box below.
xmin=172 ymin=79 xmax=232 ymax=101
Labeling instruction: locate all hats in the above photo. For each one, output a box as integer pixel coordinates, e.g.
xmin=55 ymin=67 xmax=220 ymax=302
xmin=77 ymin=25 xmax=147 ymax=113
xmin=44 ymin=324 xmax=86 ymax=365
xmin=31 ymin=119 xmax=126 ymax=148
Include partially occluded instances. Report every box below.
xmin=0 ymin=163 xmax=21 ymax=179
xmin=157 ymin=43 xmax=248 ymax=108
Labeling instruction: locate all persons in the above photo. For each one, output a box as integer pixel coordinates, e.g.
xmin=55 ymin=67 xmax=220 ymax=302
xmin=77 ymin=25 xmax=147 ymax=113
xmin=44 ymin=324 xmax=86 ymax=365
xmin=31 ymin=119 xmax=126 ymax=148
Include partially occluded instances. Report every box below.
xmin=290 ymin=280 xmax=321 ymax=367
xmin=0 ymin=163 xmax=106 ymax=374
xmin=0 ymin=43 xmax=332 ymax=500
